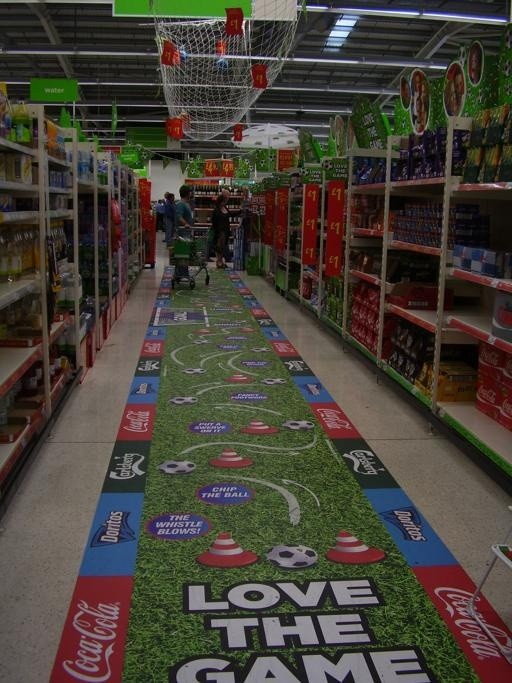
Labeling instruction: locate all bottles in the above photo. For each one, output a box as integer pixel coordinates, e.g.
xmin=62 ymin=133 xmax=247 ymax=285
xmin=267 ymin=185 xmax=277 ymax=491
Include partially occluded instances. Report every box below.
xmin=0 ymin=82 xmax=90 ymax=434
xmin=194 ymin=184 xmax=244 ymax=224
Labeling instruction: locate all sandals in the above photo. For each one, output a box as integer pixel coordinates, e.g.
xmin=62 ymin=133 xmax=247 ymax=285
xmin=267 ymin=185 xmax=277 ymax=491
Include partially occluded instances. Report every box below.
xmin=216 ymin=260 xmax=226 ymax=268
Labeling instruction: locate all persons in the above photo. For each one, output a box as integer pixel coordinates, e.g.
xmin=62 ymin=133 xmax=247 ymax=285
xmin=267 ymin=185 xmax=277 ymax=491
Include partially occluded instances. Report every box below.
xmin=210 ymin=193 xmax=237 ymax=268
xmin=165 ymin=192 xmax=178 ymax=248
xmin=153 ymin=198 xmax=161 ymax=232
xmin=161 ymin=197 xmax=166 ymax=206
xmin=158 ymin=190 xmax=171 ymax=242
xmin=174 ymin=182 xmax=194 ymax=283
xmin=202 ymin=187 xmax=232 ymax=262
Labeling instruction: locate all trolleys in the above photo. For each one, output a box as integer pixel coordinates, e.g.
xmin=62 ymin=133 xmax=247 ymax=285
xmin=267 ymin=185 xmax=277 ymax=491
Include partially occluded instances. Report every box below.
xmin=168 ymin=229 xmax=213 ymax=289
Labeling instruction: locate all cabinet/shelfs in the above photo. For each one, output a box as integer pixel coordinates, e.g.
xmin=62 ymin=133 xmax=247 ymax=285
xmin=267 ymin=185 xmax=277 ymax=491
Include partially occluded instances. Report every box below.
xmin=244 ymin=114 xmax=512 ymax=498
xmin=188 ymin=181 xmax=246 ymax=262
xmin=0 ymin=97 xmax=149 ymax=513
